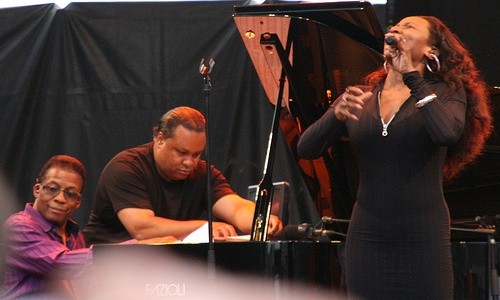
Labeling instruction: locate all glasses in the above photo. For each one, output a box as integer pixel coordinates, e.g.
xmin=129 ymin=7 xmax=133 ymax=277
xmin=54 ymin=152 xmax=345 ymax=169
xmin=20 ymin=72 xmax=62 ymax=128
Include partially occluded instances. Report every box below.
xmin=41 ymin=182 xmax=82 ymax=202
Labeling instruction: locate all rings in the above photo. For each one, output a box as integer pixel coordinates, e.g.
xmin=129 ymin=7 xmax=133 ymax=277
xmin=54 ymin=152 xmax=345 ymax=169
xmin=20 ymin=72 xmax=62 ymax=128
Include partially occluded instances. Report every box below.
xmin=346 ymin=88 xmax=349 ymax=93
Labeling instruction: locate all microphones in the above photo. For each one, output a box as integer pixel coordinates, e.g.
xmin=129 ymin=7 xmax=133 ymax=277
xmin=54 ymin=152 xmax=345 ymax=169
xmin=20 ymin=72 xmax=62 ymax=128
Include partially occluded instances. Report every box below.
xmin=284 ymin=223 xmax=347 ymax=240
xmin=386 ymin=36 xmax=396 ymax=47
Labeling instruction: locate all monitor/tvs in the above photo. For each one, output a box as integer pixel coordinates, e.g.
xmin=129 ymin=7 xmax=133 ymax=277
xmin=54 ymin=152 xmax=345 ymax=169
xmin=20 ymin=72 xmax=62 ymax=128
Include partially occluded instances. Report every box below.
xmin=247 ymin=182 xmax=290 ymax=226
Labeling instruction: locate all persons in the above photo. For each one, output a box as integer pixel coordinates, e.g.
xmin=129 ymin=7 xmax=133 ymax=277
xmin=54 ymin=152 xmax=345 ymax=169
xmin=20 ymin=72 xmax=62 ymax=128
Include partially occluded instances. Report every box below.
xmin=296 ymin=16 xmax=492 ymax=300
xmin=0 ymin=155 xmax=94 ymax=300
xmin=83 ymin=106 xmax=282 ymax=251
